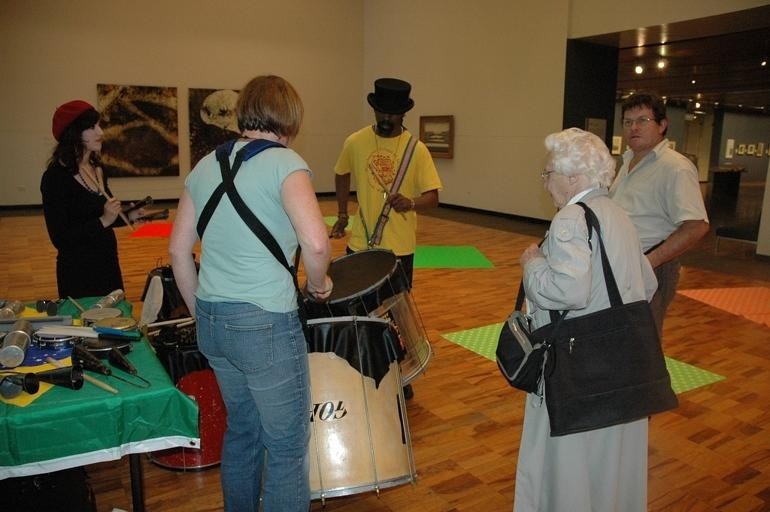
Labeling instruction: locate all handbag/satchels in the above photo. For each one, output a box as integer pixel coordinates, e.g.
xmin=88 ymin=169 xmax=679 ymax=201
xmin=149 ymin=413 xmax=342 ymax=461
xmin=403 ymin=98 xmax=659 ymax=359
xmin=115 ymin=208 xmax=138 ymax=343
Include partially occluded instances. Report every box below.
xmin=496 ymin=300 xmax=678 ymax=436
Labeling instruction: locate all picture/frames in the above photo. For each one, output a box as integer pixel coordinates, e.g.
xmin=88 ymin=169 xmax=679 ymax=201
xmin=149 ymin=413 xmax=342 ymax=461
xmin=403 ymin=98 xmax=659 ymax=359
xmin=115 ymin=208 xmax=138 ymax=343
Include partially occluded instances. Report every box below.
xmin=419 ymin=115 xmax=454 ymax=159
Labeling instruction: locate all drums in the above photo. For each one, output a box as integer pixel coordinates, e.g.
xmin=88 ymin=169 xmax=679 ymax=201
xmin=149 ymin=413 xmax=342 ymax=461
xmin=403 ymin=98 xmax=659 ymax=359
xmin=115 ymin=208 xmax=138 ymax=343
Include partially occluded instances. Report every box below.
xmin=304 ymin=317 xmax=418 ymax=507
xmin=140 ymin=262 xmax=199 ymax=318
xmin=301 ymin=249 xmax=435 ymax=385
xmin=144 ymin=315 xmax=227 ymax=470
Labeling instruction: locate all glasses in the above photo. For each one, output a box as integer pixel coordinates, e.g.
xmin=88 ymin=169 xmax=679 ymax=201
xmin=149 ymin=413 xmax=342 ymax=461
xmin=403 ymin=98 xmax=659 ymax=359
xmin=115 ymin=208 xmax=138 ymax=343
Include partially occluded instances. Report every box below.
xmin=542 ymin=169 xmax=555 ymax=180
xmin=623 ymin=116 xmax=656 ymax=126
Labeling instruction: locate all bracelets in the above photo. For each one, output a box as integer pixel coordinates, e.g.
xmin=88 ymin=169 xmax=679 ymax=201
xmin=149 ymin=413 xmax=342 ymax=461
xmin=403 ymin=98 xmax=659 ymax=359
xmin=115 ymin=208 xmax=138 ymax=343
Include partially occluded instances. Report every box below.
xmin=411 ymin=199 xmax=415 ymax=208
xmin=337 ymin=215 xmax=349 ymax=219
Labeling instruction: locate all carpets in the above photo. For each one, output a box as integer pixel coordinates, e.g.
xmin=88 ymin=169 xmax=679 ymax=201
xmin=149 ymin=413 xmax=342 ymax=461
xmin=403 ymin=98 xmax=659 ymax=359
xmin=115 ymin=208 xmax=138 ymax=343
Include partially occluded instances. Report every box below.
xmin=129 ymin=221 xmax=172 ymax=239
xmin=441 ymin=319 xmax=726 ymax=398
xmin=323 ymin=215 xmax=355 ymax=231
xmin=413 ymin=245 xmax=495 ymax=269
xmin=674 ymin=286 xmax=770 ymax=327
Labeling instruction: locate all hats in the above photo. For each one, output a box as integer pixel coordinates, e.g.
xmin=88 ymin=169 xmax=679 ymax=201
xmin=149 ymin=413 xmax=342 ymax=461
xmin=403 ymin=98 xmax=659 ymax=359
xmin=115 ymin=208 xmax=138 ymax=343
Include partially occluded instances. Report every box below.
xmin=52 ymin=100 xmax=95 ymax=142
xmin=367 ymin=78 xmax=414 ymax=115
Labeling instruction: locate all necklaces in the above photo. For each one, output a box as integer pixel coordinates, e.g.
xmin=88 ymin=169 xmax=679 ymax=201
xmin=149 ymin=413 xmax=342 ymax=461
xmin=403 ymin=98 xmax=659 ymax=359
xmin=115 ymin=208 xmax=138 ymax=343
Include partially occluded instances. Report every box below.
xmin=374 ymin=122 xmax=403 ymax=200
xmin=77 ymin=159 xmax=101 ymax=195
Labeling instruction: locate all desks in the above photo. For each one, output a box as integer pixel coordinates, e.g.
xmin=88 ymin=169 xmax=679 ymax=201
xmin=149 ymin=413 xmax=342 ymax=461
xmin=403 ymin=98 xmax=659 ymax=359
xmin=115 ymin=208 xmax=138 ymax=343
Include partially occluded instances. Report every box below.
xmin=0 ymin=296 xmax=175 ymax=512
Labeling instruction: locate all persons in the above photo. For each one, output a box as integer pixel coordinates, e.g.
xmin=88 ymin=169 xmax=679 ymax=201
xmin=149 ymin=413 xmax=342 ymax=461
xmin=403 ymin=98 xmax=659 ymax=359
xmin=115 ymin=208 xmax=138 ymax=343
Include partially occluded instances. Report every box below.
xmin=606 ymin=90 xmax=710 ymax=345
xmin=38 ymin=100 xmax=146 ymax=300
xmin=166 ymin=74 xmax=334 ymax=512
xmin=328 ymin=77 xmax=443 ymax=401
xmin=512 ymin=128 xmax=660 ymax=512
